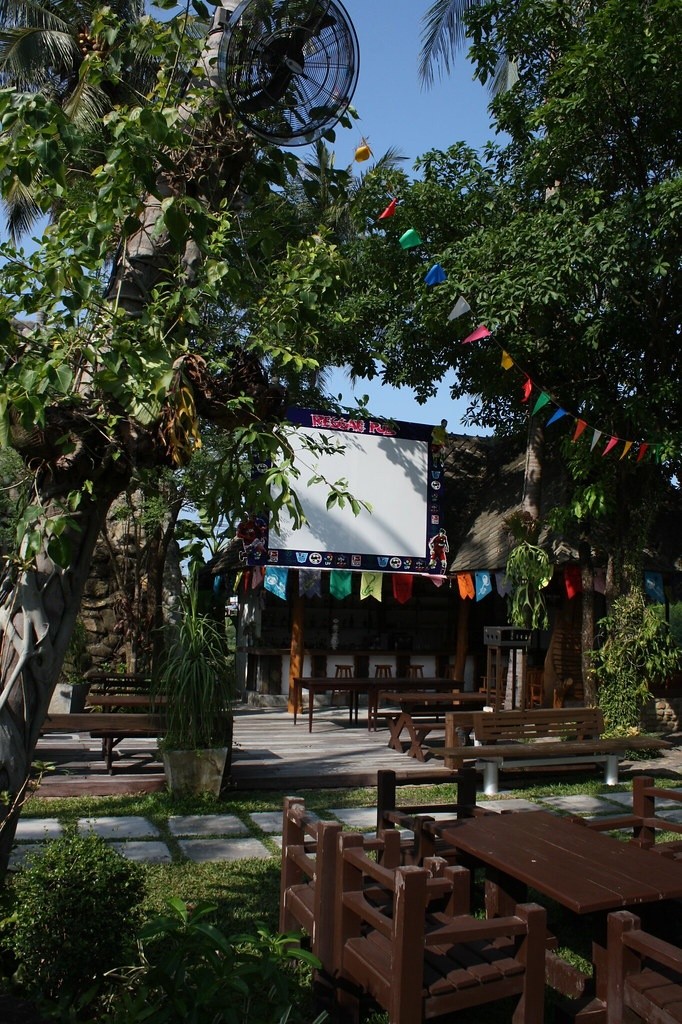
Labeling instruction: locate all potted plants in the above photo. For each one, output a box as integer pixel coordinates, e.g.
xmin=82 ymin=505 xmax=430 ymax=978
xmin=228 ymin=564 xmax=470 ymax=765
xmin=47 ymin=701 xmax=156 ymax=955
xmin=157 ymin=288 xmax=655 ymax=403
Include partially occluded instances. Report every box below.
xmin=146 ymin=564 xmax=232 ymax=806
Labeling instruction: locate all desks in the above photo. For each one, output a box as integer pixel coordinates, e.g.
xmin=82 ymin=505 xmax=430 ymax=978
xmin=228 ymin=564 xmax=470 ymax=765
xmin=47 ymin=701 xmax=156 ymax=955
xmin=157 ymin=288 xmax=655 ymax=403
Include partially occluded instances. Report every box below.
xmin=486 ymin=645 xmax=527 ymax=712
xmin=40 ymin=670 xmax=232 ymax=775
xmin=424 ymin=810 xmax=682 ymax=1024
xmin=294 ymin=678 xmax=504 ymax=762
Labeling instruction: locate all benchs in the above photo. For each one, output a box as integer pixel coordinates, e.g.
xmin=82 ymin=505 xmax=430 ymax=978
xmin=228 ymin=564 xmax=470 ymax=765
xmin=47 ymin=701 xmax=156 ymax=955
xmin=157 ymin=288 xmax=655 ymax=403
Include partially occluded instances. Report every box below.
xmin=369 ymin=712 xmax=445 ymax=718
xmin=429 ymin=710 xmax=673 ymax=792
xmin=89 ymin=730 xmax=175 ymax=739
xmin=90 ymin=688 xmax=151 ymax=696
xmin=405 ymin=723 xmax=445 ymax=732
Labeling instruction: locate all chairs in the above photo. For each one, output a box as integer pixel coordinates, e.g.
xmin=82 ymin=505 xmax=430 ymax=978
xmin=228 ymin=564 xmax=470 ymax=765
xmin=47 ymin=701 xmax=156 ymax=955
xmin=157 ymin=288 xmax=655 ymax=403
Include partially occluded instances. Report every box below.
xmin=279 ymin=795 xmax=446 ymax=989
xmin=565 ymin=776 xmax=682 ymax=863
xmin=531 ymin=672 xmax=544 ymax=709
xmin=334 ymin=830 xmax=546 ymax=1023
xmin=435 ymin=664 xmax=455 ymax=705
xmin=377 ymin=768 xmax=514 ymax=869
xmin=330 ymin=664 xmax=356 ymax=707
xmin=607 ymin=910 xmax=682 ymax=1024
xmin=407 ymin=665 xmax=425 ymax=692
xmin=374 ymin=664 xmax=393 ymax=705
